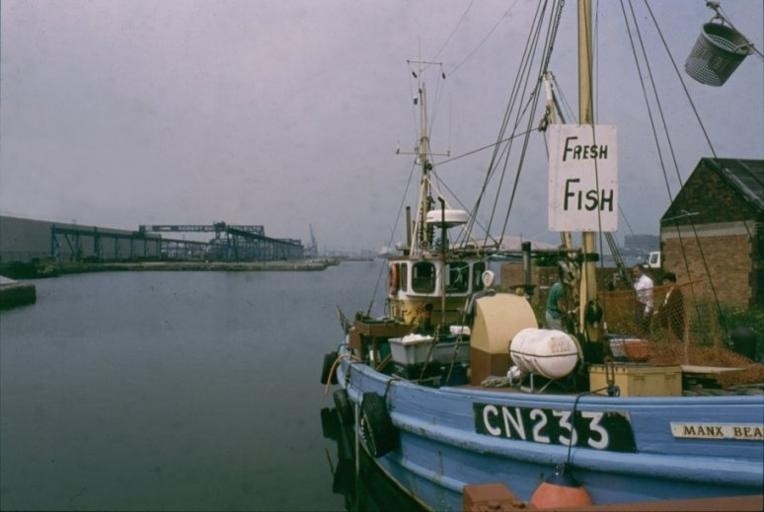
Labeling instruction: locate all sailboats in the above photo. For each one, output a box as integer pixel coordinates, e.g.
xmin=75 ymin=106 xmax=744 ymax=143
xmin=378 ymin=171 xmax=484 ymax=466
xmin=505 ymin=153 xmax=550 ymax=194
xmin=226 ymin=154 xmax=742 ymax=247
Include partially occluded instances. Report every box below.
xmin=320 ymin=0 xmax=764 ymax=512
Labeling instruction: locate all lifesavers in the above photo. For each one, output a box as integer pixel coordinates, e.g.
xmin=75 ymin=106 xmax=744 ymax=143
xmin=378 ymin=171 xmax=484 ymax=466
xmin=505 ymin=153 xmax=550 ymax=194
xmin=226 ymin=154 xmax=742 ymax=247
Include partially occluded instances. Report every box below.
xmin=389 ymin=264 xmax=397 ymax=295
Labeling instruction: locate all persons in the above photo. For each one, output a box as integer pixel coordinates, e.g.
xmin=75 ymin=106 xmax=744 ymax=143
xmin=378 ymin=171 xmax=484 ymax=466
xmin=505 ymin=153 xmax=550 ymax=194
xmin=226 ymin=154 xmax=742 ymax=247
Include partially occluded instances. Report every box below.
xmin=652 ymin=272 xmax=685 ymax=342
xmin=630 ymin=264 xmax=654 ymax=338
xmin=416 ymin=303 xmax=436 ymax=336
xmin=543 ymin=266 xmax=577 ymax=334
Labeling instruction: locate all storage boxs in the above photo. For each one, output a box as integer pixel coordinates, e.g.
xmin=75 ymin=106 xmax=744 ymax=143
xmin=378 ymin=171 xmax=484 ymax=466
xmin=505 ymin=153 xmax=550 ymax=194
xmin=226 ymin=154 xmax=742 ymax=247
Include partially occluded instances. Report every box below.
xmin=588 ymin=365 xmax=682 ymax=397
xmin=388 ymin=337 xmax=434 ymax=365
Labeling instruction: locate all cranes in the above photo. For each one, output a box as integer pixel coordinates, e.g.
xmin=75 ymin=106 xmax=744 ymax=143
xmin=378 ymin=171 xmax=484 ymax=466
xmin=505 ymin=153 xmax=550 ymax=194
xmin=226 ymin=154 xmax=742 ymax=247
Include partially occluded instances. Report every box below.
xmin=139 ymin=220 xmax=264 ymax=262
xmin=305 ymin=223 xmax=318 ymax=260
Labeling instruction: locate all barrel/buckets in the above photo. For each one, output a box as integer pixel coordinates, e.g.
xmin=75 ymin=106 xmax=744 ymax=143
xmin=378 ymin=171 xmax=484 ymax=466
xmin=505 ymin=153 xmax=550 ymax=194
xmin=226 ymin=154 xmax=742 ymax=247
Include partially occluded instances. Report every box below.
xmin=511 ymin=327 xmax=579 ymax=378
